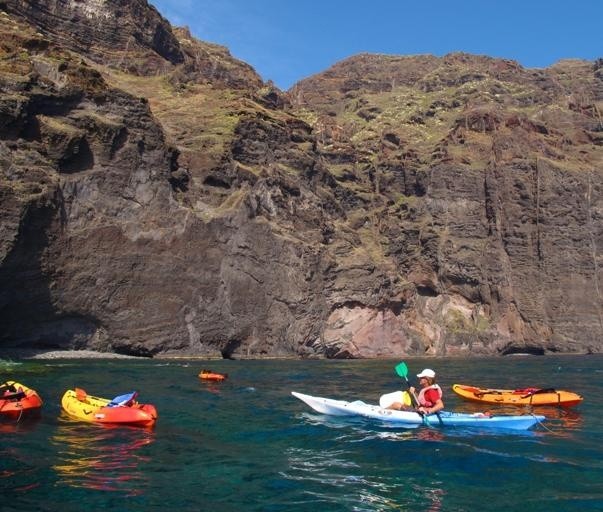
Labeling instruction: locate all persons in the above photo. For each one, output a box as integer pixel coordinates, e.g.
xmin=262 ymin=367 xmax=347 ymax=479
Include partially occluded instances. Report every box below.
xmin=388 ymin=368 xmax=445 ymax=415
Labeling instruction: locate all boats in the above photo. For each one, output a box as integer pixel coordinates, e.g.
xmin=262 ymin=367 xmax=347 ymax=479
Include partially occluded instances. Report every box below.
xmin=452 ymin=382 xmax=584 ymax=408
xmin=0 ymin=380 xmax=42 ymax=419
xmin=199 ymin=373 xmax=225 ymax=381
xmin=290 ymin=390 xmax=547 ymax=433
xmin=61 ymin=389 xmax=158 ymax=427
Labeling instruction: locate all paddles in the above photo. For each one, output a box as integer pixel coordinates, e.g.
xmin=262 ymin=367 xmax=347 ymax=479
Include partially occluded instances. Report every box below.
xmin=395 ymin=362 xmax=431 ymax=426
xmin=75 ymin=388 xmax=109 ymax=404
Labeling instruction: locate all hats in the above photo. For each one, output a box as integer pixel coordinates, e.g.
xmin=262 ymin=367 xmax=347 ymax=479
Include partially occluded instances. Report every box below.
xmin=416 ymin=369 xmax=435 ymax=377
